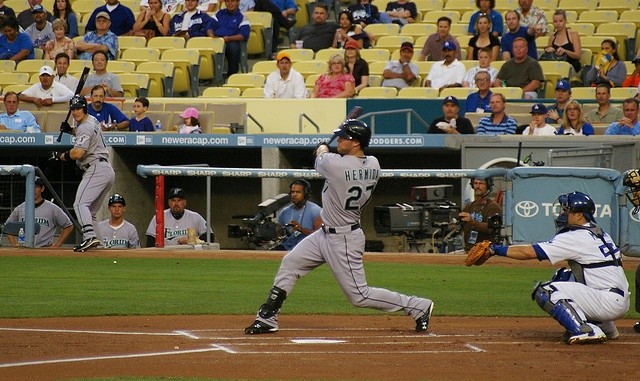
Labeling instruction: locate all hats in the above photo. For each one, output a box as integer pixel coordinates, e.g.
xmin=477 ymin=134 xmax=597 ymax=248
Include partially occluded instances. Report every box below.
xmin=277 ymin=52 xmax=291 ymax=61
xmin=96 ymin=11 xmax=111 ymax=20
xmin=34 ymin=174 xmax=43 ymax=185
xmin=167 ymin=187 xmax=186 ymax=199
xmin=179 ymin=108 xmax=199 ymax=119
xmin=32 ymin=4 xmax=46 ymax=14
xmin=38 ymin=65 xmax=54 ymax=76
xmin=442 ymin=41 xmax=456 ymax=51
xmin=555 ymin=80 xmax=570 ymax=90
xmin=443 ymin=96 xmax=458 ymax=106
xmin=529 ymin=103 xmax=547 ymax=114
xmin=345 ymin=41 xmax=358 ymax=49
xmin=401 ymin=42 xmax=413 ymax=51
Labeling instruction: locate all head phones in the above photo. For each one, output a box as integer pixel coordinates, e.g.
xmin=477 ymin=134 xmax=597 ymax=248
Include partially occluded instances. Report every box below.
xmin=289 ymin=178 xmax=313 ymax=200
xmin=470 ymin=175 xmax=495 ymax=190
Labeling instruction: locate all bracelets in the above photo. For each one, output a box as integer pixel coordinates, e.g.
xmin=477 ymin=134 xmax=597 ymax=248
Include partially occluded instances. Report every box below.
xmin=64 ymin=150 xmax=73 ymax=162
xmin=403 ymin=61 xmax=408 ymax=65
xmin=492 ymin=245 xmax=509 ymax=256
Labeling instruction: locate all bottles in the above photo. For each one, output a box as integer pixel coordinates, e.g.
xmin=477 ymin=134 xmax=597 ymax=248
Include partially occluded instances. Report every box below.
xmin=18 ymin=227 xmax=25 ymax=247
xmin=96 ymin=113 xmax=101 ymax=125
xmin=154 ymin=119 xmax=162 ymax=132
xmin=112 ymin=120 xmax=118 ymax=132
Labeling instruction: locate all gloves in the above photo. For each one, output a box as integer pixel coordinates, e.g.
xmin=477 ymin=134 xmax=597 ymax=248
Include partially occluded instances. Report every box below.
xmin=48 ymin=150 xmax=63 ymax=161
xmin=60 ymin=120 xmax=73 ymax=134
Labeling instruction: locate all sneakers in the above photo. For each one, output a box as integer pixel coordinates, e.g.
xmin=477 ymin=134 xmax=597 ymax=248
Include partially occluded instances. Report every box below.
xmin=567 ymin=322 xmax=608 ymax=345
xmin=415 ymin=301 xmax=435 ymax=332
xmin=594 ymin=321 xmax=620 ymax=340
xmin=74 ymin=237 xmax=100 ymax=252
xmin=245 ymin=315 xmax=279 ymax=335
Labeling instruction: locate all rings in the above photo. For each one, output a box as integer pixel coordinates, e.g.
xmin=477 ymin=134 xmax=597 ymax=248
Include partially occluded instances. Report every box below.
xmin=296 ymin=225 xmax=298 ymax=227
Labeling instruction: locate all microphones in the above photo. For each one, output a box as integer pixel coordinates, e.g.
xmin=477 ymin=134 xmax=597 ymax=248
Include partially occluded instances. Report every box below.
xmin=298 ymin=203 xmax=302 ymax=208
xmin=481 ymin=193 xmax=484 ymax=199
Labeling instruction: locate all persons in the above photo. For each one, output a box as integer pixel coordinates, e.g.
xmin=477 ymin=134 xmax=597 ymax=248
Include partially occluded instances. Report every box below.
xmin=204 ymin=0 xmax=251 ymax=76
xmin=545 ymin=79 xmax=572 ymax=124
xmin=493 ymin=37 xmax=544 ymax=100
xmin=98 ymin=194 xmax=140 ymax=249
xmin=468 ymin=0 xmax=504 ymax=37
xmin=179 ymin=108 xmax=202 ymax=134
xmin=0 ymin=0 xmax=15 ymax=19
xmin=133 ymin=0 xmax=220 ymax=40
xmin=465 ymin=192 xmax=631 ymax=345
xmin=263 ymin=53 xmax=307 ymax=99
xmin=419 ymin=17 xmax=461 ymax=61
xmin=462 ymin=48 xmax=498 ymax=88
xmin=312 ymin=54 xmax=355 ymax=98
xmin=381 ymin=42 xmax=419 ymax=89
xmin=49 ymin=0 xmax=78 ymax=39
xmin=146 ymin=188 xmax=214 ymax=247
xmin=0 ymin=18 xmax=35 ymax=65
xmin=244 ymin=120 xmax=434 ymax=335
xmin=76 ymin=11 xmax=119 ymax=61
xmin=343 ymin=41 xmax=370 ymax=94
xmin=586 ymin=40 xmax=627 ymax=87
xmin=238 ymin=0 xmax=380 ymax=53
xmin=522 ymin=104 xmax=558 ymax=136
xmin=87 ymin=86 xmax=130 ymax=131
xmin=385 ymin=0 xmax=418 ymax=23
xmin=605 ymin=98 xmax=640 ymax=135
xmin=558 ymin=101 xmax=595 ymax=136
xmin=448 ymin=177 xmax=503 ymax=254
xmin=272 ymin=179 xmax=322 ymax=250
xmin=583 ymin=84 xmax=625 ymax=124
xmin=539 ymin=11 xmax=582 ymax=72
xmin=129 ymin=97 xmax=155 ymax=131
xmin=476 ymin=94 xmax=518 ymax=135
xmin=18 ymin=65 xmax=74 ymax=107
xmin=85 ymin=0 xmax=135 ymax=37
xmin=500 ymin=10 xmax=543 ymax=61
xmin=4 ymin=175 xmax=74 ymax=248
xmin=622 ymin=56 xmax=640 ymax=87
xmin=0 ymin=91 xmax=42 ymax=133
xmin=17 ymin=0 xmax=52 ymax=30
xmin=514 ymin=0 xmax=549 ymax=35
xmin=424 ymin=41 xmax=466 ymax=88
xmin=80 ymin=50 xmax=123 ymax=97
xmin=463 ymin=71 xmax=493 ymax=113
xmin=44 ymin=18 xmax=77 ymax=59
xmin=427 ymin=96 xmax=474 ymax=134
xmin=468 ymin=15 xmax=499 ymax=61
xmin=24 ymin=4 xmax=56 ymax=50
xmin=48 ymin=95 xmax=116 ymax=252
xmin=54 ymin=53 xmax=79 ymax=92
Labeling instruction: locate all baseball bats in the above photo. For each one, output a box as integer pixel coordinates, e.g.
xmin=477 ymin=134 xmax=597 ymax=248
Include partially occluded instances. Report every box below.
xmin=327 ymin=105 xmax=364 ymax=146
xmin=57 ymin=67 xmax=91 ymax=142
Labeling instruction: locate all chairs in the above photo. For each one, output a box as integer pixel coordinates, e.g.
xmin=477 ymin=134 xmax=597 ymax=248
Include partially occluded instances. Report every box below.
xmin=165 ymin=102 xmax=206 ymax=112
xmin=173 ymin=111 xmax=212 ymax=134
xmin=597 ymin=0 xmax=638 ymax=11
xmin=397 ymin=86 xmax=438 ymax=99
xmin=459 ymin=10 xmax=506 ymax=23
xmin=374 ymin=34 xmax=415 ymax=48
xmin=45 ymin=109 xmax=75 ymax=133
xmin=556 ymin=0 xmax=598 ymax=10
xmin=276 ymin=48 xmax=313 ymax=61
xmin=620 ymin=10 xmax=639 ymax=28
xmin=508 ymin=112 xmax=532 ymax=125
xmin=198 ymin=86 xmax=241 ymax=99
xmin=546 ymin=23 xmax=596 ymax=35
xmin=356 ymin=87 xmax=397 ymax=99
xmin=415 ymin=37 xmax=430 ymax=47
xmin=242 ymin=88 xmax=264 ymax=97
xmin=161 ymin=48 xmax=200 ymax=92
xmin=34 ymin=46 xmax=44 ymax=59
xmin=504 ymin=101 xmax=555 ymax=114
xmin=370 ymin=0 xmax=444 ymax=23
xmin=113 ymin=74 xmax=149 ymax=97
xmin=85 ymin=100 xmax=121 ymax=111
xmin=539 ymin=61 xmax=571 ymax=99
xmin=1 ymin=60 xmax=15 ymax=71
xmin=136 ymin=61 xmax=175 ymax=97
xmin=17 ymin=60 xmax=57 ymax=73
xmin=315 ymin=48 xmax=390 ymax=61
xmin=46 ymin=100 xmax=73 ymax=110
xmin=457 ymin=35 xmax=475 ymax=47
xmin=577 ymin=35 xmax=617 ymax=49
xmin=590 ymin=124 xmax=612 ymax=132
xmin=596 ymin=23 xmax=636 ymax=60
xmin=1 ymin=98 xmax=39 ymax=111
xmin=80 ymin=16 xmax=141 ymax=31
xmin=391 ymin=48 xmax=469 ymax=61
xmin=252 ymin=61 xmax=278 ymax=73
xmin=55 ymin=60 xmax=134 ymax=74
xmin=123 ymin=99 xmax=164 ymax=111
xmin=400 ymin=24 xmax=475 ymax=36
xmin=241 ymin=11 xmax=272 ymax=54
xmin=1 ymin=72 xmax=31 ymax=83
xmin=31 ymin=74 xmax=52 ymax=82
xmin=582 ymin=103 xmax=627 ymax=119
xmin=363 ymin=25 xmax=399 ymax=40
xmin=2 ymin=84 xmax=32 ymax=94
xmin=207 ymin=102 xmax=246 ymax=127
xmin=73 ymin=35 xmax=147 ymax=47
xmin=227 ymin=74 xmax=264 ymax=87
xmin=493 ymin=0 xmax=557 ymax=11
xmin=534 ymin=35 xmax=550 ymax=49
xmin=120 ymin=46 xmax=162 ymax=62
xmin=579 ymin=10 xmax=618 ymax=23
xmin=536 ymin=49 xmax=592 ymax=66
xmin=292 ymin=62 xmax=330 ymax=75
xmin=121 ymin=111 xmax=170 ymax=130
xmin=439 ymin=88 xmax=525 ymax=100
xmin=147 ymin=35 xmax=185 ymax=48
xmin=553 ymin=122 xmax=563 ymax=132
xmin=541 ymin=11 xmax=578 ymax=23
xmin=30 ymin=110 xmax=46 ymax=131
xmin=460 ymin=60 xmax=507 ymax=71
xmin=305 ymin=75 xmax=320 ymax=90
xmin=167 ymin=10 xmax=220 ymax=20
xmin=368 ymin=61 xmax=435 ymax=85
xmin=186 ymin=36 xmax=224 ymax=80
xmin=464 ymin=112 xmax=489 ymax=125
xmin=444 ymin=0 xmax=482 ymax=11
xmin=424 ymin=10 xmax=462 ymax=23
xmin=413 ymin=76 xmax=422 ymax=85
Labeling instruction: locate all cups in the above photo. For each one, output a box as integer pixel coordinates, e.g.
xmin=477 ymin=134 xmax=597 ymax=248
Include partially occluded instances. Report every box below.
xmin=295 ymin=40 xmax=304 ymax=49
xmin=336 ymin=28 xmax=344 ymax=43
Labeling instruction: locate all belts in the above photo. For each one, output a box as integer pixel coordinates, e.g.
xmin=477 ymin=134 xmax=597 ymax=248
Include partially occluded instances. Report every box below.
xmin=610 ymin=286 xmax=625 ymax=296
xmin=85 ymin=158 xmax=108 ymax=168
xmin=321 ymin=222 xmax=360 ymax=234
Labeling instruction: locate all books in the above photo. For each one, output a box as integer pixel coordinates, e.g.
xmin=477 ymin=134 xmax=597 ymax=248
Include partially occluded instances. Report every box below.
xmin=595 ymin=50 xmax=614 ymax=76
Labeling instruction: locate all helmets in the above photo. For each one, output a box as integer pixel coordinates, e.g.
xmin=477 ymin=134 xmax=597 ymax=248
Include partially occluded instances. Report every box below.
xmin=333 ymin=119 xmax=371 ymax=148
xmin=622 ymin=169 xmax=640 ymax=215
xmin=552 ymin=191 xmax=597 ymax=234
xmin=69 ymin=95 xmax=87 ymax=114
xmin=108 ymin=193 xmax=126 ymax=207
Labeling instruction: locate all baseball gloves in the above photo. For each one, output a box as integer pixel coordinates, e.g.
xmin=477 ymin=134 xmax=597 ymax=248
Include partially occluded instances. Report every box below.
xmin=465 ymin=239 xmax=494 ymax=267
